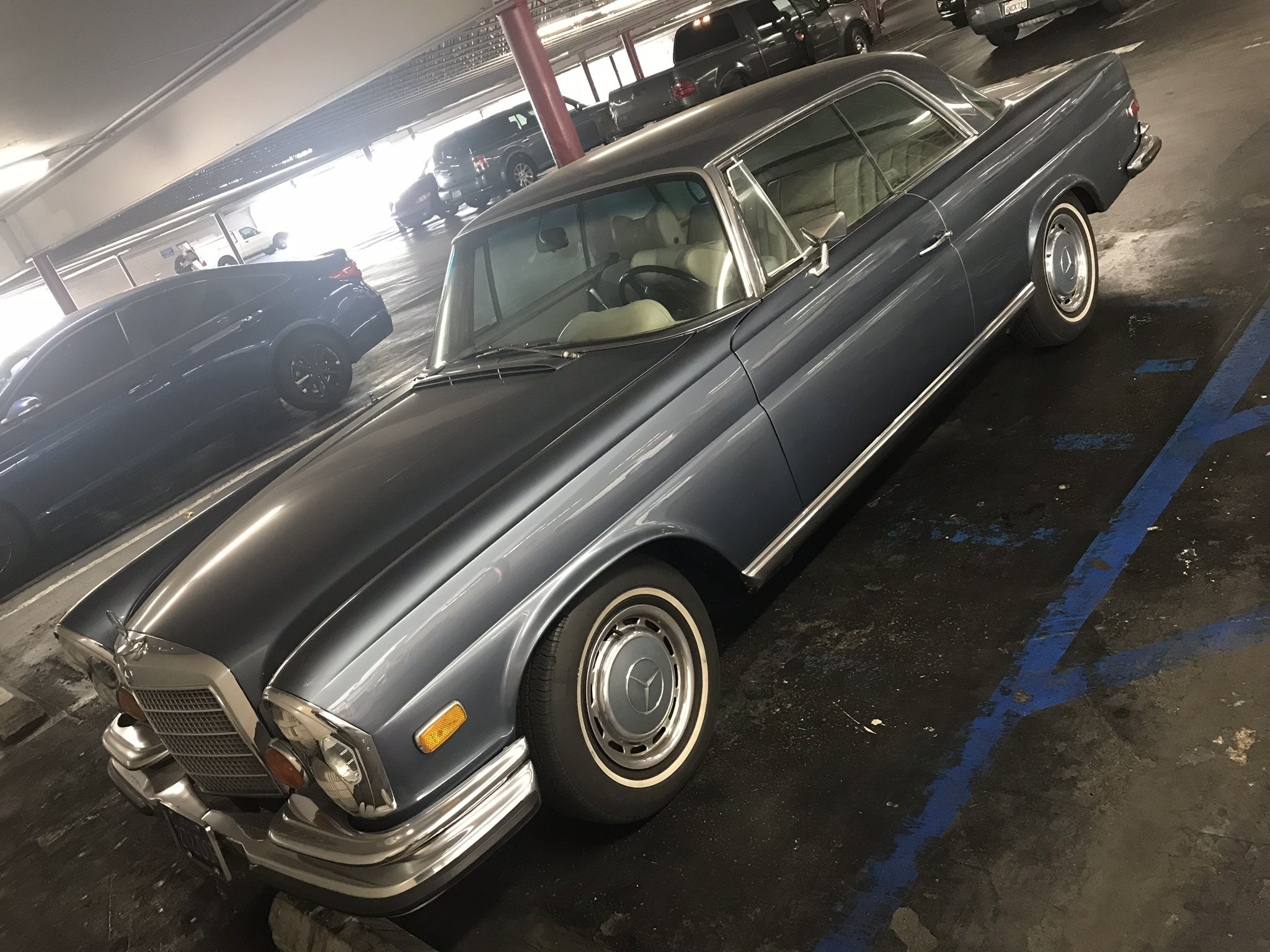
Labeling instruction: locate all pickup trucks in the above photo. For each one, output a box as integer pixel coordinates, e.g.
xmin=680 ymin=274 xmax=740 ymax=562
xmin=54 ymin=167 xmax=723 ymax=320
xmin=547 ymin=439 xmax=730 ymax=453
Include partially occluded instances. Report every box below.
xmin=392 ymin=0 xmax=887 ymax=231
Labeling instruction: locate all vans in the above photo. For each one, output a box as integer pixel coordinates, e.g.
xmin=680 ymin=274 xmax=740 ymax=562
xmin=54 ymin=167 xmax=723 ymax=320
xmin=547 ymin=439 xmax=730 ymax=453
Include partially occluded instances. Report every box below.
xmin=186 ymin=226 xmax=291 ymax=271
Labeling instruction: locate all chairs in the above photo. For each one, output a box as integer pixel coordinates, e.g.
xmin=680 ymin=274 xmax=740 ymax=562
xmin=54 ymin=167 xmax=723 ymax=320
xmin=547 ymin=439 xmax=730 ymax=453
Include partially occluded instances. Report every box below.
xmin=679 ymin=198 xmax=777 ymax=307
xmin=596 ymin=202 xmax=686 ymax=310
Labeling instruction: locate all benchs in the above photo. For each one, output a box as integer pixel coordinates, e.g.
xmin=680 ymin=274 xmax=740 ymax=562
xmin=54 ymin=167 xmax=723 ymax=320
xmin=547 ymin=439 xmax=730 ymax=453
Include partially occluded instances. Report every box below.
xmin=737 ymin=143 xmax=935 ymax=259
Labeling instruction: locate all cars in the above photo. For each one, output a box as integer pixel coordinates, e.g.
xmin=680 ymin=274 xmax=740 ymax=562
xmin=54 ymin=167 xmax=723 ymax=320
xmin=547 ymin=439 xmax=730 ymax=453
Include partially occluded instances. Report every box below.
xmin=0 ymin=248 xmax=395 ymax=599
xmin=933 ymin=1 xmax=1137 ymax=49
xmin=50 ymin=49 xmax=1166 ymax=922
